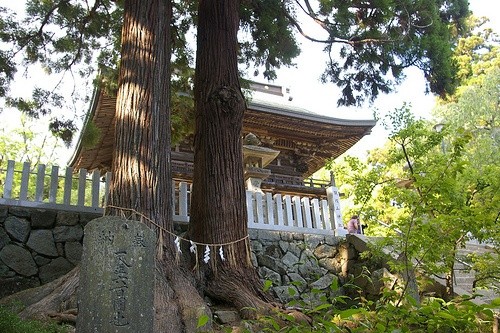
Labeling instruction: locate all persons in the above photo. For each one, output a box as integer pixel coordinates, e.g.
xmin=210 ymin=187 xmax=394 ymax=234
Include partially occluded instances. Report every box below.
xmin=348 ymin=215 xmax=358 ymax=233
xmin=357 ymin=216 xmax=368 ymax=234
